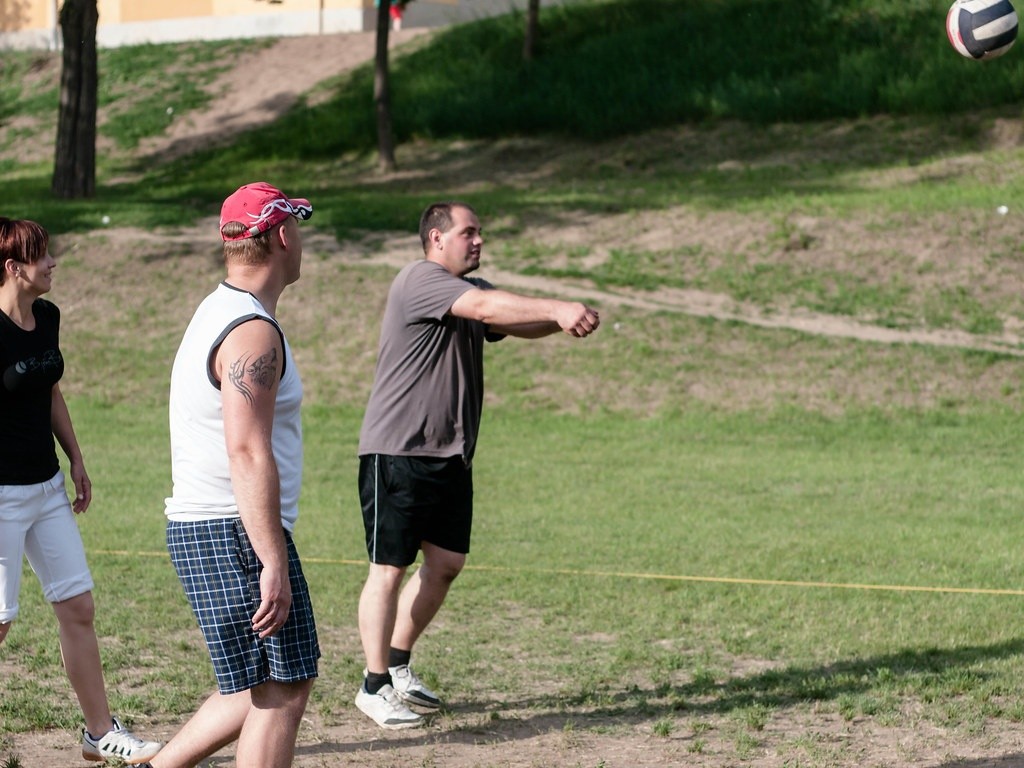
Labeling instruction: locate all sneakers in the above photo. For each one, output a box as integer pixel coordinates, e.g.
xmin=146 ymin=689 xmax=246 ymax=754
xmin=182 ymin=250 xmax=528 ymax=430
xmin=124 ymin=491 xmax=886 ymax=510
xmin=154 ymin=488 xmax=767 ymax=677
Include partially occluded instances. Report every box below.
xmin=362 ymin=663 xmax=441 ymax=714
xmin=354 ymin=678 xmax=426 ymax=730
xmin=82 ymin=716 xmax=163 ymax=766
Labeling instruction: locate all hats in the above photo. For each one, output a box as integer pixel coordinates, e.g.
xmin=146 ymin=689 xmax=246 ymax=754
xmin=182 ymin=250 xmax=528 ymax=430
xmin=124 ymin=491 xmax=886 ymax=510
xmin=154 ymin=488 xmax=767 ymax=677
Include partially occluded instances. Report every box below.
xmin=220 ymin=182 xmax=312 ymax=241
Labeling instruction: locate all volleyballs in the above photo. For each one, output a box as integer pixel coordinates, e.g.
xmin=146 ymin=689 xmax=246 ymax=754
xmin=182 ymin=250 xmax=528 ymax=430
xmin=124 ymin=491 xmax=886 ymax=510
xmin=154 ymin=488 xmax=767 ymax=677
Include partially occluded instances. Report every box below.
xmin=945 ymin=0 xmax=1019 ymax=61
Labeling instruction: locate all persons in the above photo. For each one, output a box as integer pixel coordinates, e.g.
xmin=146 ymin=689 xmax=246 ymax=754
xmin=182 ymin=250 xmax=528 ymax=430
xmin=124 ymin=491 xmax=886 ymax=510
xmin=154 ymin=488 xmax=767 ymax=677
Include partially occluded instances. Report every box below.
xmin=389 ymin=0 xmax=408 ymax=30
xmin=0 ymin=213 xmax=162 ymax=764
xmin=132 ymin=183 xmax=322 ymax=768
xmin=356 ymin=203 xmax=601 ymax=730
